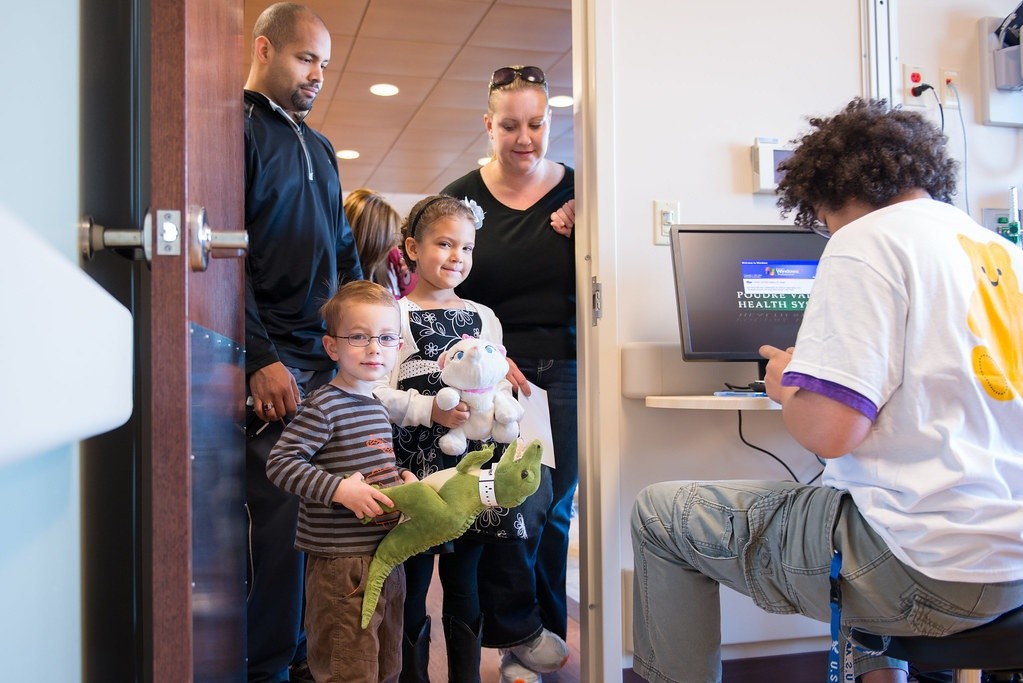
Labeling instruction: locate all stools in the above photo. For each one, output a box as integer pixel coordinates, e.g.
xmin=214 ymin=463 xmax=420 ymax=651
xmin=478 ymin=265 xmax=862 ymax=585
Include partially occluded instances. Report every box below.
xmin=849 ymin=607 xmax=1023 ymax=683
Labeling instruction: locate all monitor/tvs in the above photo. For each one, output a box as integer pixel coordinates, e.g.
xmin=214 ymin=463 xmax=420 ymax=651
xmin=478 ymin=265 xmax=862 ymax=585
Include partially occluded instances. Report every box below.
xmin=670 ymin=224 xmax=832 ymax=381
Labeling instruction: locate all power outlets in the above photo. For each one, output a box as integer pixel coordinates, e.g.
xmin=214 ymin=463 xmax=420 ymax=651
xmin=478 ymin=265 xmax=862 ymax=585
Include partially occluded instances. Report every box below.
xmin=903 ymin=61 xmax=929 ymax=109
xmin=939 ymin=66 xmax=960 ymax=109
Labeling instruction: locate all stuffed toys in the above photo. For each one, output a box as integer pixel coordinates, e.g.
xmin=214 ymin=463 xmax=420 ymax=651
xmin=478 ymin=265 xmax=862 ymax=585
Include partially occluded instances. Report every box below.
xmin=437 ymin=333 xmax=521 ymax=456
xmin=342 ymin=439 xmax=543 ymax=630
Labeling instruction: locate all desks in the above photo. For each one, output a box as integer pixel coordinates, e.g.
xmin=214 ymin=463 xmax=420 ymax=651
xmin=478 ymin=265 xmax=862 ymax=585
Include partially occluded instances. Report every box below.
xmin=645 ymin=394 xmax=783 ymax=411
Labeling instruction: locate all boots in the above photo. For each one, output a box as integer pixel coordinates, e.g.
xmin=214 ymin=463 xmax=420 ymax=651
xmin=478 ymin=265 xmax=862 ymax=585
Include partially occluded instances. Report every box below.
xmin=442 ymin=614 xmax=484 ymax=683
xmin=399 ymin=615 xmax=431 ymax=683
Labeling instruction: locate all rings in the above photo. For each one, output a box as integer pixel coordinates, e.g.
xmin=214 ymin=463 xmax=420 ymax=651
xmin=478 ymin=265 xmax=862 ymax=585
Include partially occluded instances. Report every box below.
xmin=264 ymin=404 xmax=272 ymax=411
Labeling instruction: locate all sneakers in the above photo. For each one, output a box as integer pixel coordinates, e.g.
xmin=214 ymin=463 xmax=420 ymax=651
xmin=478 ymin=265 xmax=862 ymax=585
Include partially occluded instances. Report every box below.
xmin=508 ymin=626 xmax=569 ymax=674
xmin=496 ymin=646 xmax=542 ymax=683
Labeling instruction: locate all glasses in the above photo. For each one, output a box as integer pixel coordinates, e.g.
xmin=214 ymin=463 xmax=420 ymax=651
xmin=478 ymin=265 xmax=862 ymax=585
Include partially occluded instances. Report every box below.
xmin=489 ymin=65 xmax=547 ymax=93
xmin=331 ymin=332 xmax=405 ymax=347
xmin=810 ymin=190 xmax=832 ymax=240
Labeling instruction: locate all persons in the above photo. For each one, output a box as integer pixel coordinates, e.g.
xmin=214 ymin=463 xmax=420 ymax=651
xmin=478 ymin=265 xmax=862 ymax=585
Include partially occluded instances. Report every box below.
xmin=265 ymin=282 xmax=420 ymax=683
xmin=344 ymin=189 xmax=403 ymax=281
xmin=631 ymin=100 xmax=1023 ymax=683
xmin=373 ymin=193 xmax=504 ymax=683
xmin=438 ymin=65 xmax=576 ymax=683
xmin=243 ymin=3 xmax=364 ymax=683
